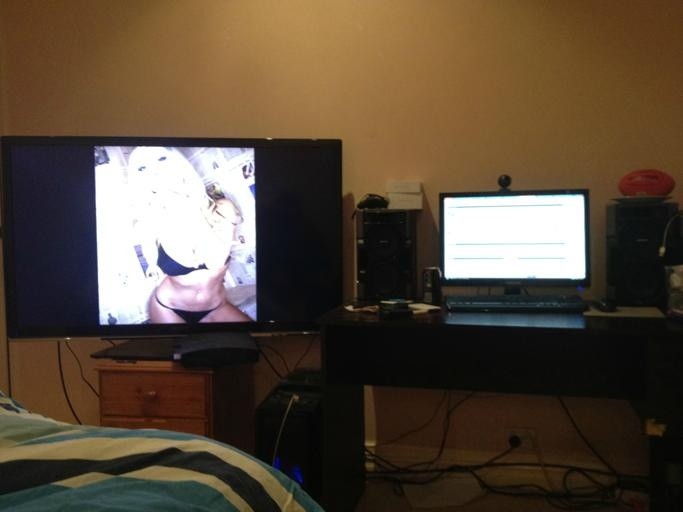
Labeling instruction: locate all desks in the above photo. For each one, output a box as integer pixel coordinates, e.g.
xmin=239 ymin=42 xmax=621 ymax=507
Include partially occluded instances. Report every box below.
xmin=318 ymin=302 xmax=681 ymax=485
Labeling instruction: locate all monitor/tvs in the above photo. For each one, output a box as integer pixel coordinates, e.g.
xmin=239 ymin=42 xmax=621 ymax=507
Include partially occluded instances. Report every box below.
xmin=1 ymin=135 xmax=342 ymax=361
xmin=439 ymin=187 xmax=591 ymax=294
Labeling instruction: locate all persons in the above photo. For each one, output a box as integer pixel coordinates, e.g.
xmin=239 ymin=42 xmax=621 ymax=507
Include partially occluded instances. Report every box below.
xmin=124 ymin=147 xmax=253 ymax=325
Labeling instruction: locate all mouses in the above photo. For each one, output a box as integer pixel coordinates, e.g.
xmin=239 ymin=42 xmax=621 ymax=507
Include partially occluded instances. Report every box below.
xmin=595 ymin=296 xmax=616 ymax=312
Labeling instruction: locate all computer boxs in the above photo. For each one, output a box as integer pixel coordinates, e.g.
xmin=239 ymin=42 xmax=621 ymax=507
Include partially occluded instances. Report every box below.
xmin=251 ymin=367 xmax=322 ymax=501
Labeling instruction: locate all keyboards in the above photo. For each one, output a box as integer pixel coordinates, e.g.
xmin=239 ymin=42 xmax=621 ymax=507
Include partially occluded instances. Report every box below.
xmin=445 ymin=295 xmax=584 ymax=315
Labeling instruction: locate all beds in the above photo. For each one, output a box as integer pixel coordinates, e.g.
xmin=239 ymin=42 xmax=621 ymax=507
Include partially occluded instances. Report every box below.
xmin=0 ymin=389 xmax=328 ymax=511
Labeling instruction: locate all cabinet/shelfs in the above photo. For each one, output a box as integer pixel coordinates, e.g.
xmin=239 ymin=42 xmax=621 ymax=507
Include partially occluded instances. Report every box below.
xmin=90 ymin=363 xmax=251 ymax=454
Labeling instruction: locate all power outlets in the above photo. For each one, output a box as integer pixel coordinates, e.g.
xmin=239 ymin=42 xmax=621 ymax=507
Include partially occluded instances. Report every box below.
xmin=502 ymin=427 xmax=538 ymax=453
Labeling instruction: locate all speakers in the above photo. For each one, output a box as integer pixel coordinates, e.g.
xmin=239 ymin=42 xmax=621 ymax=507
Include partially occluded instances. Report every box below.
xmin=606 ymin=203 xmax=677 ymax=306
xmin=353 ymin=208 xmax=419 ymax=305
